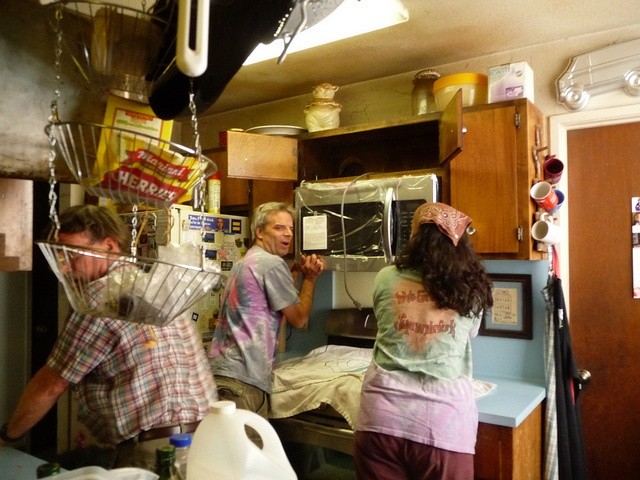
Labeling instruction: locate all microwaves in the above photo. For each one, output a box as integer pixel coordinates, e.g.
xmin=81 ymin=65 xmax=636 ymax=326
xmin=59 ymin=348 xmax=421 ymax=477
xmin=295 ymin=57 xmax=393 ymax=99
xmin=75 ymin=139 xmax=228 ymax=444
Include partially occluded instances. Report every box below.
xmin=294 ymin=175 xmax=438 ymax=272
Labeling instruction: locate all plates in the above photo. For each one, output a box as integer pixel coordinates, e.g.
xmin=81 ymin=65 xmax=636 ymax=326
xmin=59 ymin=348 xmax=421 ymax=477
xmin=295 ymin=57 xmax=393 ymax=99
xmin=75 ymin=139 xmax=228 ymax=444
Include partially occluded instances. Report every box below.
xmin=245 ymin=125 xmax=306 ymax=136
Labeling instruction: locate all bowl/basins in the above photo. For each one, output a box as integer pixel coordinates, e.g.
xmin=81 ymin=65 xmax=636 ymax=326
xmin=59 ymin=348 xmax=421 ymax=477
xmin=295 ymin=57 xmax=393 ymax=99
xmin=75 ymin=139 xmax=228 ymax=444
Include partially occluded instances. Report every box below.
xmin=432 ymin=72 xmax=487 ymax=111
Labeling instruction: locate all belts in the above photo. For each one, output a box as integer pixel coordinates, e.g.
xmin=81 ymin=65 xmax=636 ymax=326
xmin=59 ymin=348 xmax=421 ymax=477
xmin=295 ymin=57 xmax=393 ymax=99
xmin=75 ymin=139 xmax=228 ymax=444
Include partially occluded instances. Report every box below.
xmin=115 ymin=419 xmax=201 ymax=448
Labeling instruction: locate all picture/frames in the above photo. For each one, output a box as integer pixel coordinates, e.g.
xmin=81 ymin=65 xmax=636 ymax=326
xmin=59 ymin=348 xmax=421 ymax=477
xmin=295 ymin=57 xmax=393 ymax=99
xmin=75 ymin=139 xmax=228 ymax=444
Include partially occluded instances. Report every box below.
xmin=480 ymin=275 xmax=533 ymax=340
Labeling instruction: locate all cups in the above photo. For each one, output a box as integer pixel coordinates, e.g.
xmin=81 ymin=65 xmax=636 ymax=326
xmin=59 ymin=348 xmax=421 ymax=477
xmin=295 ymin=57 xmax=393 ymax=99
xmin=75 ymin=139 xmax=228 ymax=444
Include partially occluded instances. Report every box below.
xmin=530 ymin=181 xmax=559 ymax=211
xmin=544 ymin=155 xmax=564 ymax=184
xmin=547 ymin=189 xmax=564 ymax=213
xmin=532 ymin=213 xmax=561 ymax=244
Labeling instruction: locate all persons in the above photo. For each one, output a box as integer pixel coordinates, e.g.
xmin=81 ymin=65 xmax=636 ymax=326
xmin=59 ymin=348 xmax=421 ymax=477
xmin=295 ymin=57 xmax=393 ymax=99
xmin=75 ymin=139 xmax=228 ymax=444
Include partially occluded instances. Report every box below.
xmin=352 ymin=202 xmax=492 ymax=480
xmin=0 ymin=203 xmax=219 ymax=480
xmin=208 ymin=200 xmax=325 ymax=451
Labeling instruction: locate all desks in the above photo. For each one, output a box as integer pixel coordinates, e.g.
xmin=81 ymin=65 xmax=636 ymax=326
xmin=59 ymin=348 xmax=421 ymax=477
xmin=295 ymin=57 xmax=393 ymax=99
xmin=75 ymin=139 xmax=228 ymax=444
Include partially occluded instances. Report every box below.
xmin=269 ymin=349 xmax=547 ymax=477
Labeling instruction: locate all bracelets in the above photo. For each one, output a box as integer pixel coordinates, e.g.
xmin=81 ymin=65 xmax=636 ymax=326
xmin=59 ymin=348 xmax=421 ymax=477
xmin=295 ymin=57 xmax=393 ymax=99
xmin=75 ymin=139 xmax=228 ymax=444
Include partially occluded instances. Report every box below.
xmin=0 ymin=421 xmax=23 ymax=445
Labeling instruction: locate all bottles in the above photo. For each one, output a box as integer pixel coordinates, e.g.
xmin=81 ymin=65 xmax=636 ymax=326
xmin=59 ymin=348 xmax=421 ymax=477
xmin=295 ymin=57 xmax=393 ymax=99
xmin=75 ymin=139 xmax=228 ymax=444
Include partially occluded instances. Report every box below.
xmin=152 ymin=445 xmax=181 ymax=480
xmin=412 ymin=79 xmax=435 ymax=115
xmin=169 ymin=434 xmax=192 ymax=480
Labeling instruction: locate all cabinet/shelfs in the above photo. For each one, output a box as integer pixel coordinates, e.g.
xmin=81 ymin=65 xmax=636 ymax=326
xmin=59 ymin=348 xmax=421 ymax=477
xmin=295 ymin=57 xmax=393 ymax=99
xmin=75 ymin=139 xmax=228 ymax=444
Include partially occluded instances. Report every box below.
xmin=250 ymin=181 xmax=293 ymax=259
xmin=179 ymin=146 xmax=250 ymax=212
xmin=226 ymin=91 xmax=465 ymax=271
xmin=449 ymin=98 xmax=543 ymax=260
